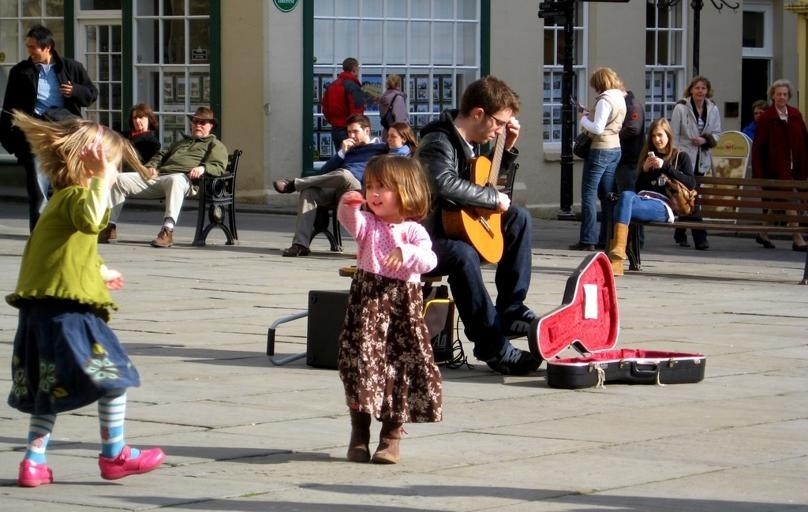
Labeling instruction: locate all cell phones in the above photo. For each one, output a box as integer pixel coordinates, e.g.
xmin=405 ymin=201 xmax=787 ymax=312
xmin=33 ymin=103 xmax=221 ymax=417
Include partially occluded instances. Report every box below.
xmin=647 ymin=151 xmax=655 ymax=158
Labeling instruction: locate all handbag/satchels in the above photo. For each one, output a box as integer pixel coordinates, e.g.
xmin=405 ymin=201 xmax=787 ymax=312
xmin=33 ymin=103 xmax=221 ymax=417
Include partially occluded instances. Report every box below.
xmin=665 ymin=151 xmax=696 ymax=215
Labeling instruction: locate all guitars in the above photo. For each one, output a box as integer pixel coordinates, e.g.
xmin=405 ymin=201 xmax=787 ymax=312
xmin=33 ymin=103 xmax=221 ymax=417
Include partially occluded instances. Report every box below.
xmin=440 ymin=114 xmax=509 ymax=267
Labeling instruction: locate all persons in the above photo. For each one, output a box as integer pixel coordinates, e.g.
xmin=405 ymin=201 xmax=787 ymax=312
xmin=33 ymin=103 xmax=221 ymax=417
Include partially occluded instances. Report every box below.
xmin=323 ymin=58 xmax=366 ymax=155
xmin=120 ymin=105 xmax=162 ymax=173
xmin=741 ymin=100 xmax=770 ymax=141
xmin=7 ymin=109 xmax=165 ymax=487
xmin=384 ymin=123 xmax=417 ymax=157
xmin=414 ymin=76 xmax=543 ymax=376
xmin=602 ymin=118 xmax=696 ymax=276
xmin=379 ymin=75 xmax=409 ymax=143
xmin=336 ymin=156 xmax=442 ymax=464
xmin=273 ymin=115 xmax=388 ymax=257
xmin=670 ymin=76 xmax=721 ymax=250
xmin=569 ymin=67 xmax=627 ymax=252
xmin=751 ymin=79 xmax=808 ymax=252
xmin=98 ymin=107 xmax=228 ymax=248
xmin=610 ymin=89 xmax=646 ymax=251
xmin=0 ymin=27 xmax=98 ymax=235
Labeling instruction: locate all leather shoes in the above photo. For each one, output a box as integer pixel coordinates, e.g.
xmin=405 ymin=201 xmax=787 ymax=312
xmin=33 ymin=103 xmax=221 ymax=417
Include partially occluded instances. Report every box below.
xmin=674 ymin=234 xmax=690 ymax=248
xmin=99 ymin=447 xmax=164 ymax=479
xmin=792 ymin=244 xmax=807 ymax=251
xmin=100 ymin=224 xmax=118 ymax=244
xmin=697 ymin=239 xmax=710 ymax=251
xmin=284 ymin=243 xmax=309 ymax=256
xmin=756 ymin=233 xmax=776 ymax=249
xmin=150 ymin=226 xmax=175 ymax=249
xmin=19 ymin=460 xmax=54 ymax=488
xmin=273 ymin=178 xmax=299 ymax=194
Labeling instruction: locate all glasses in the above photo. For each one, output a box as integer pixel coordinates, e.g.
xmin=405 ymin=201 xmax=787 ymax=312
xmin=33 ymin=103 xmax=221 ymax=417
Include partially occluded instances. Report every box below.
xmin=190 ymin=121 xmax=212 ymax=126
xmin=486 ymin=112 xmax=508 ymax=128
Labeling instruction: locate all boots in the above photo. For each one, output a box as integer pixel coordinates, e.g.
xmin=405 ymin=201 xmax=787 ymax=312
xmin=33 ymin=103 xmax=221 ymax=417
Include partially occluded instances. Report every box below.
xmin=346 ymin=407 xmax=370 ymax=461
xmin=372 ymin=420 xmax=406 ymax=464
xmin=607 ymin=241 xmax=624 ymax=277
xmin=609 ymin=223 xmax=628 ymax=261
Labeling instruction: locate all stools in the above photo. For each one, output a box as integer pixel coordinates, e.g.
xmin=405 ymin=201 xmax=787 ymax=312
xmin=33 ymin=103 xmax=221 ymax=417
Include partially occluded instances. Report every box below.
xmin=340 ymin=267 xmax=441 ymax=286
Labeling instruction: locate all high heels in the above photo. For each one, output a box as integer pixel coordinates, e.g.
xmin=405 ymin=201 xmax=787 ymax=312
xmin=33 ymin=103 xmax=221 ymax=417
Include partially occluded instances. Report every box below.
xmin=570 ymin=242 xmax=597 ymax=251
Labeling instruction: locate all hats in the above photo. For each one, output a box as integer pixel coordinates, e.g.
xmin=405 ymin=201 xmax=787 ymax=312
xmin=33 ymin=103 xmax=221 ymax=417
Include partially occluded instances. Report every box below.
xmin=187 ymin=107 xmax=218 ymax=124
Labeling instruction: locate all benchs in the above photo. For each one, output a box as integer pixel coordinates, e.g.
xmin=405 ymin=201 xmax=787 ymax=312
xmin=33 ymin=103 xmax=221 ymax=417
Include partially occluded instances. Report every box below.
xmin=192 ymin=148 xmax=243 ymax=247
xmin=309 ymin=155 xmax=520 ymax=252
xmin=606 ymin=177 xmax=808 ymax=285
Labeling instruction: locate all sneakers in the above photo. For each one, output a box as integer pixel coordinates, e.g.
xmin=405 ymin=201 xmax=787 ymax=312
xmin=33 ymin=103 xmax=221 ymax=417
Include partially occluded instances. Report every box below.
xmin=488 ymin=349 xmax=543 ymax=377
xmin=499 ymin=304 xmax=538 ymax=343
